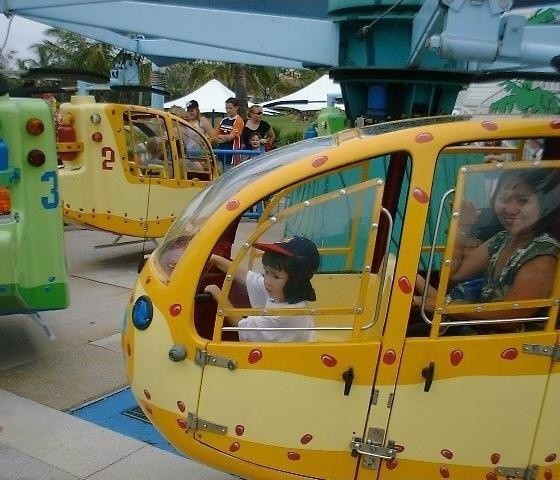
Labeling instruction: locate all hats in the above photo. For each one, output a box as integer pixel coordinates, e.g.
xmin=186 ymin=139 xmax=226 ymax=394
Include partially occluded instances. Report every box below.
xmin=252 ymin=236 xmax=320 ymax=271
xmin=186 ymin=100 xmax=198 ymax=108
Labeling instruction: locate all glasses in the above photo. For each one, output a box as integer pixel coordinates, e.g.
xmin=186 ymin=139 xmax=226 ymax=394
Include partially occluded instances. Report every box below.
xmin=252 ymin=112 xmax=262 ymax=114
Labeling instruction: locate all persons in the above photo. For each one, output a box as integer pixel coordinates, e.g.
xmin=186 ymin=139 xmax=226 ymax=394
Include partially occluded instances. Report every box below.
xmin=142 ymin=96 xmax=275 ymax=178
xmin=204 ymin=234 xmax=321 ymax=345
xmin=406 ymin=167 xmax=560 ymax=337
